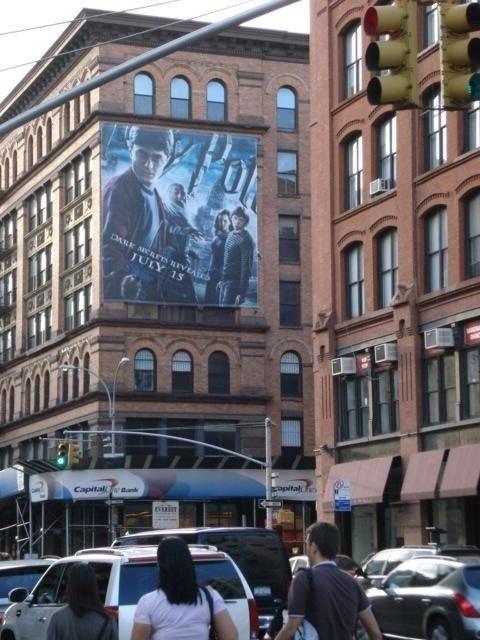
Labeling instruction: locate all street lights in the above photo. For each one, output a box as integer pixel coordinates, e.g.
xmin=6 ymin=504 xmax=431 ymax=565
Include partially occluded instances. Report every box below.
xmin=57 ymin=355 xmax=132 ymax=454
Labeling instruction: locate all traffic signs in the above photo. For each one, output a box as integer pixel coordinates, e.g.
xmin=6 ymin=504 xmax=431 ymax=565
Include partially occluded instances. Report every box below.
xmin=105 ymin=498 xmax=125 ymax=507
xmin=258 ymin=499 xmax=284 ymax=509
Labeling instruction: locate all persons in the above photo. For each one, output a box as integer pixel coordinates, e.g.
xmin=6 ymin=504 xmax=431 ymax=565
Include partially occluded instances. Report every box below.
xmin=43 ymin=559 xmax=117 ymax=638
xmin=130 ymin=539 xmax=237 ymax=640
xmin=274 ymin=519 xmax=382 ymax=640
xmin=102 ymin=124 xmax=255 ymax=306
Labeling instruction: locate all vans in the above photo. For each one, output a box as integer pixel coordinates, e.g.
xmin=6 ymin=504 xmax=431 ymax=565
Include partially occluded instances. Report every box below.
xmin=112 ymin=525 xmax=294 ymax=640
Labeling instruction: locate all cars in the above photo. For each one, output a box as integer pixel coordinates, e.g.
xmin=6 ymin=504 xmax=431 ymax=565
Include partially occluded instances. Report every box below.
xmin=286 ymin=553 xmax=359 ymax=583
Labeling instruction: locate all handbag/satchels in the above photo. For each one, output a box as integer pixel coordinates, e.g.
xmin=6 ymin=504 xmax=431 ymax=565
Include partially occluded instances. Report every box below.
xmin=267 ymin=608 xmax=320 ymax=640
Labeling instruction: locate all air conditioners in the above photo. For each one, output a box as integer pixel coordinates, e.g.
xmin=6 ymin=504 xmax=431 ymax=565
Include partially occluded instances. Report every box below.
xmin=375 ymin=344 xmax=397 ymax=364
xmin=369 ymin=178 xmax=386 ymax=196
xmin=331 ymin=356 xmax=357 ymax=376
xmin=424 ymin=327 xmax=454 ymax=350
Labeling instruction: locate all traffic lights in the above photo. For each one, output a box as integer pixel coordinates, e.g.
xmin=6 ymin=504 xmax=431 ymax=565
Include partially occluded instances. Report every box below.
xmin=56 ymin=442 xmax=68 ymax=466
xmin=437 ymin=0 xmax=479 ymax=114
xmin=363 ymin=0 xmax=421 ymax=114
xmin=69 ymin=442 xmax=81 ymax=465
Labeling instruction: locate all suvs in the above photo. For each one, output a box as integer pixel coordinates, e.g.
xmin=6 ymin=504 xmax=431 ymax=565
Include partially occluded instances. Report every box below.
xmin=363 ymin=552 xmax=480 ymax=640
xmin=0 ymin=542 xmax=261 ymax=640
xmin=350 ymin=541 xmax=480 ymax=588
xmin=0 ymin=553 xmax=65 ymax=625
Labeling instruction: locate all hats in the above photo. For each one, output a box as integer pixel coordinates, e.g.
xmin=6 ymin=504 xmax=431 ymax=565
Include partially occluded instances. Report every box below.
xmin=129 ymin=126 xmax=174 ymax=157
xmin=336 ymin=558 xmax=359 ymax=573
